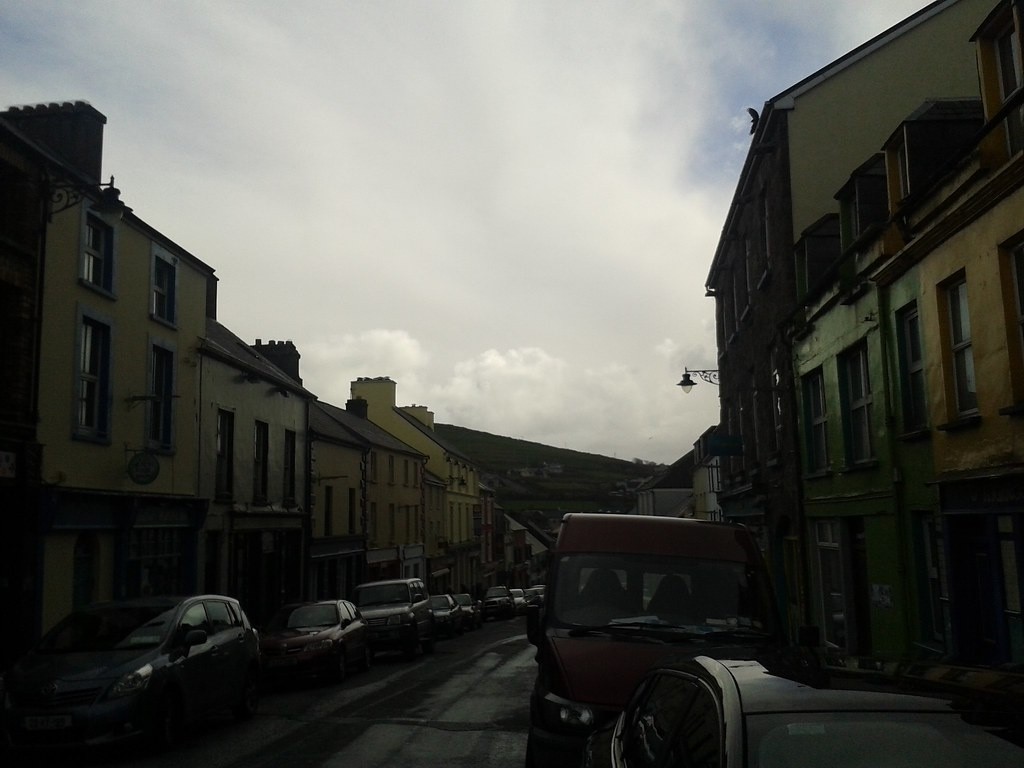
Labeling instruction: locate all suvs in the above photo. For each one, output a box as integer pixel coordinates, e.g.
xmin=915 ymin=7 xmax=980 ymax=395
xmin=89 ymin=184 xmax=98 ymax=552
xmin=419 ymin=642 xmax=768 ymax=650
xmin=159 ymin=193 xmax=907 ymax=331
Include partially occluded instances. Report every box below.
xmin=23 ymin=594 xmax=261 ymax=763
xmin=478 ymin=585 xmax=515 ymax=622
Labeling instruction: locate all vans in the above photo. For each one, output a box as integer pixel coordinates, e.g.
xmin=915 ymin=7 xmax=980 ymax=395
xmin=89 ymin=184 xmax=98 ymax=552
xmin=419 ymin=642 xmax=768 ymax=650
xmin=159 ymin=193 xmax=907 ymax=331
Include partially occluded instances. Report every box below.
xmin=523 ymin=509 xmax=826 ymax=768
xmin=348 ymin=578 xmax=435 ymax=653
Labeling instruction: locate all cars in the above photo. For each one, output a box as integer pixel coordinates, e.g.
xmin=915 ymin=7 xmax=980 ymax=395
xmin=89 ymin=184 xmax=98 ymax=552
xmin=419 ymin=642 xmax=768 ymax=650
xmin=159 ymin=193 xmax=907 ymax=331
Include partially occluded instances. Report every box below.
xmin=430 ymin=594 xmax=462 ymax=636
xmin=524 ymin=588 xmax=541 ymax=607
xmin=533 ymin=587 xmax=544 ymax=604
xmin=509 ymin=589 xmax=528 ymax=616
xmin=597 ymin=653 xmax=1023 ymax=768
xmin=260 ymin=597 xmax=378 ymax=691
xmin=452 ymin=593 xmax=482 ymax=630
